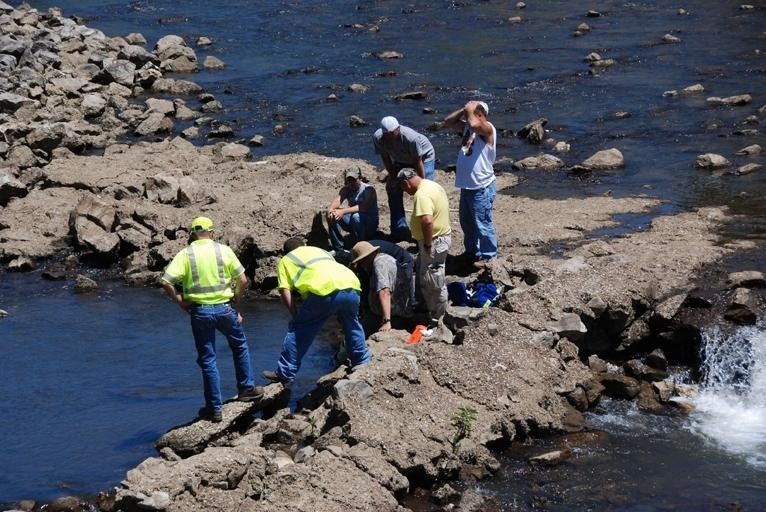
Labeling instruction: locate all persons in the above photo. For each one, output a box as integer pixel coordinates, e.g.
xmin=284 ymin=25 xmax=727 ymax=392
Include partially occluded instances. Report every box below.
xmin=369 ymin=113 xmax=436 ymax=233
xmin=325 ymin=164 xmax=380 ymax=249
xmin=444 ymin=100 xmax=499 ymax=265
xmin=332 ymin=239 xmax=412 ymax=371
xmin=334 ymin=237 xmax=415 ymax=268
xmin=396 ymin=167 xmax=453 ymax=329
xmin=259 ymin=235 xmax=372 ymax=384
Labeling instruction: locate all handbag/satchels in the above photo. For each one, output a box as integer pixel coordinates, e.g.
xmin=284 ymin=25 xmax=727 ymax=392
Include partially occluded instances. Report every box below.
xmin=446 ymin=280 xmax=497 ymax=308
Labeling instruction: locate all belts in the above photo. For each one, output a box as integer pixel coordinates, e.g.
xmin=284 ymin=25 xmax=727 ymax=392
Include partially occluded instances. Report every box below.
xmin=194 ymin=302 xmax=231 ymax=308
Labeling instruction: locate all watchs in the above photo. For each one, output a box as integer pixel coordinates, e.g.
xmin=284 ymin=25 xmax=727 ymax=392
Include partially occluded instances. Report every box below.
xmin=379 ymin=313 xmax=393 ymax=327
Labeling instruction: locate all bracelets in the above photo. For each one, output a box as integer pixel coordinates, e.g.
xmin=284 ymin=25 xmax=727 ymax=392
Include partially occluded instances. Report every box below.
xmin=158 ymin=216 xmax=265 ymax=422
xmin=423 ymin=244 xmax=431 ymax=249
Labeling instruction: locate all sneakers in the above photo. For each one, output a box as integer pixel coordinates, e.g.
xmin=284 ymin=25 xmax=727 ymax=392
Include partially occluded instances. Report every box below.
xmin=262 ymin=371 xmax=288 ymax=383
xmin=198 ymin=407 xmax=222 ymax=422
xmin=337 ymin=352 xmax=356 ymax=363
xmin=238 ymin=385 xmax=264 ymax=402
xmin=457 ymin=254 xmax=468 ymax=261
xmin=345 ymin=166 xmax=361 ymax=180
xmin=473 ymin=259 xmax=489 ymax=267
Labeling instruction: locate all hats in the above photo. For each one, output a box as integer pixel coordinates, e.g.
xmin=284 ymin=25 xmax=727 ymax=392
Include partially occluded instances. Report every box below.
xmin=381 ymin=116 xmax=399 ymax=133
xmin=192 ymin=216 xmax=213 ymax=232
xmin=478 ymin=102 xmax=489 ymax=114
xmin=395 ymin=168 xmax=416 ymax=181
xmin=352 ymin=241 xmax=380 ymax=263
